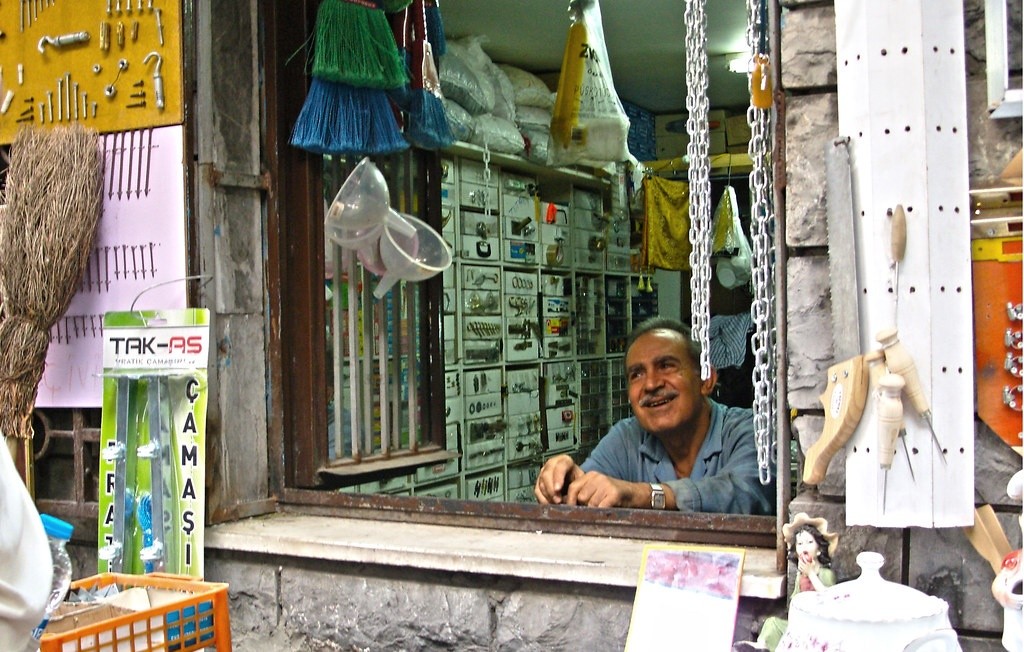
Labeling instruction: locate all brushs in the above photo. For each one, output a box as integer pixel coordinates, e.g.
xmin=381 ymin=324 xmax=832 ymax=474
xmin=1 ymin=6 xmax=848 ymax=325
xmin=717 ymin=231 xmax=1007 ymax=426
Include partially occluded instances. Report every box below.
xmin=286 ymin=0 xmax=457 ymax=159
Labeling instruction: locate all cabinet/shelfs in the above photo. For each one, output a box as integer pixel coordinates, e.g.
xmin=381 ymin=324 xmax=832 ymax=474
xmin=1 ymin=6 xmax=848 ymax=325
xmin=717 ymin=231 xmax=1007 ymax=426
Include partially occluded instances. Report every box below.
xmin=325 ymin=141 xmax=661 ymax=507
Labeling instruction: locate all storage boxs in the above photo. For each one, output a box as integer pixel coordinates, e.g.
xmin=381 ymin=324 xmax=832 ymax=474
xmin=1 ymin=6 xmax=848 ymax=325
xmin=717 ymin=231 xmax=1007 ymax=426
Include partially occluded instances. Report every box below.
xmin=34 ymin=571 xmax=233 ymax=652
xmin=620 ymin=98 xmax=752 ymax=159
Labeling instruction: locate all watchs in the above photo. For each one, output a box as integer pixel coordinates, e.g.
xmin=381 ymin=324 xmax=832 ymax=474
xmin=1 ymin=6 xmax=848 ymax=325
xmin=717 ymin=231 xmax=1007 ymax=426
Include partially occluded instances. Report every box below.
xmin=647 ymin=482 xmax=666 ymax=510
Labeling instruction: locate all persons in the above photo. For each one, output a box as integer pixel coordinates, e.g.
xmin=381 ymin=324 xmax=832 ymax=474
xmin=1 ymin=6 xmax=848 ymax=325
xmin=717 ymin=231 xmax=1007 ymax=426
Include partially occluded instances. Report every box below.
xmin=0 ymin=427 xmax=56 ymax=652
xmin=532 ymin=316 xmax=777 ymax=517
xmin=752 ymin=512 xmax=841 ymax=652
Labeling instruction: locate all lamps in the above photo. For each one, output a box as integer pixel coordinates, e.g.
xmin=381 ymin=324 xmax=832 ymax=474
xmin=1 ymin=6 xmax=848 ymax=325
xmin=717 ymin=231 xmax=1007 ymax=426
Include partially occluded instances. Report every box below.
xmin=725 ymin=53 xmax=751 ymax=74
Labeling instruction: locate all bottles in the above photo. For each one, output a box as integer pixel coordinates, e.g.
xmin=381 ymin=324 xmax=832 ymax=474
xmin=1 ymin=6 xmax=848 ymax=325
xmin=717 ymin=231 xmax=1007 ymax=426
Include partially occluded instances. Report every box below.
xmin=25 ymin=513 xmax=73 ymax=652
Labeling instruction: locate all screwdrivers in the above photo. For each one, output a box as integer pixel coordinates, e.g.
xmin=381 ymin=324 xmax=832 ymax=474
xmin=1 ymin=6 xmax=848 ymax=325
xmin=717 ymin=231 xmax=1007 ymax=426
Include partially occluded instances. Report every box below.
xmin=889 ymin=205 xmax=908 ymax=328
xmin=876 ymin=330 xmax=948 ymax=468
xmin=875 ymin=374 xmax=907 ymax=516
xmin=865 ymin=349 xmax=915 ymax=484
